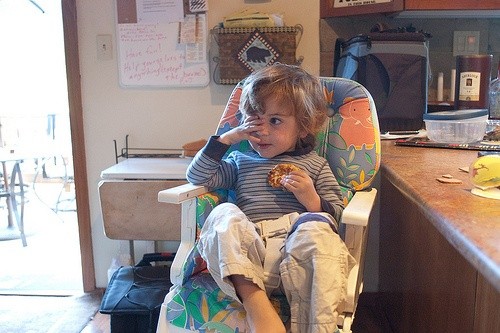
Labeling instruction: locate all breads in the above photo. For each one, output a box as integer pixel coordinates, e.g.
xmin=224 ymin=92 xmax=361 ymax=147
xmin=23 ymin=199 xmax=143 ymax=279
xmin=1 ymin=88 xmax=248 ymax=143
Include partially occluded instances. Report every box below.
xmin=266 ymin=164 xmax=299 ymax=188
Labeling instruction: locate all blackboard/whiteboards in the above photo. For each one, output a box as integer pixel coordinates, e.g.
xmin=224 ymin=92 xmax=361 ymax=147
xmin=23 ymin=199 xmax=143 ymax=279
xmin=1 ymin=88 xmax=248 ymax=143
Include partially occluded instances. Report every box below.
xmin=114 ymin=0 xmax=210 ymax=88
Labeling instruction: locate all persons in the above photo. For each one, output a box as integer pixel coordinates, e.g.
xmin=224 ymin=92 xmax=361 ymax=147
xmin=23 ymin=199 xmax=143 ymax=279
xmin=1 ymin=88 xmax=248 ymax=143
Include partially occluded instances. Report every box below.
xmin=186 ymin=61 xmax=357 ymax=333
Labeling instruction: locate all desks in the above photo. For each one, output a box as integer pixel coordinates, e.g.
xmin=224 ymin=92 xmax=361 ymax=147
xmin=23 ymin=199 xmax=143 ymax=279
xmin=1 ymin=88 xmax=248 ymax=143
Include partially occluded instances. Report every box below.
xmin=101 ymin=157 xmax=194 ymax=180
xmin=377 ymin=127 xmax=500 ymax=333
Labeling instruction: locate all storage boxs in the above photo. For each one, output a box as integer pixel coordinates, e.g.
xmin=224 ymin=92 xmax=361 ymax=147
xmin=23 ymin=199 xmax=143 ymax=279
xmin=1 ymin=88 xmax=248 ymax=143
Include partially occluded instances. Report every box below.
xmin=333 ymin=22 xmax=433 ymax=131
xmin=210 ymin=23 xmax=304 ymax=85
xmin=423 ymin=109 xmax=489 ymax=143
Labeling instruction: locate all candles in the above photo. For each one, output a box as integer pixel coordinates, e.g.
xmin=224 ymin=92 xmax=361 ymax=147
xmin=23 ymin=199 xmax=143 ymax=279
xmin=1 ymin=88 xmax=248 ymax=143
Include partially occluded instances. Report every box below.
xmin=438 ymin=66 xmax=456 ymax=102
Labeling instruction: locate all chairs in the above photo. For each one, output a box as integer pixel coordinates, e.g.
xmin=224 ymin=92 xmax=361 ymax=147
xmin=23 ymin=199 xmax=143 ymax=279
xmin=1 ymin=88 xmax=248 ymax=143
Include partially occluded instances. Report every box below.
xmin=158 ymin=76 xmax=381 ymax=333
xmin=99 ymin=182 xmax=189 ymax=267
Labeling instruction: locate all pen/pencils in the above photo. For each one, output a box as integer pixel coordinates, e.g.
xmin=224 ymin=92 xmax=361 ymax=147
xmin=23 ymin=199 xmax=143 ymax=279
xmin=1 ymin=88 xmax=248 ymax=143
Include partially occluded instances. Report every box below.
xmin=385 ymin=131 xmax=419 ymax=134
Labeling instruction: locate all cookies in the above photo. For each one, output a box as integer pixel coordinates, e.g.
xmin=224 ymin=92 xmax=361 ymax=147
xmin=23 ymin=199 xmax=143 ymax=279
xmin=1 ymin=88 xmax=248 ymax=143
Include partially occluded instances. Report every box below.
xmin=442 ymin=174 xmax=453 ymax=178
xmin=435 ymin=178 xmax=462 ymax=184
xmin=458 ymin=167 xmax=470 ymax=173
